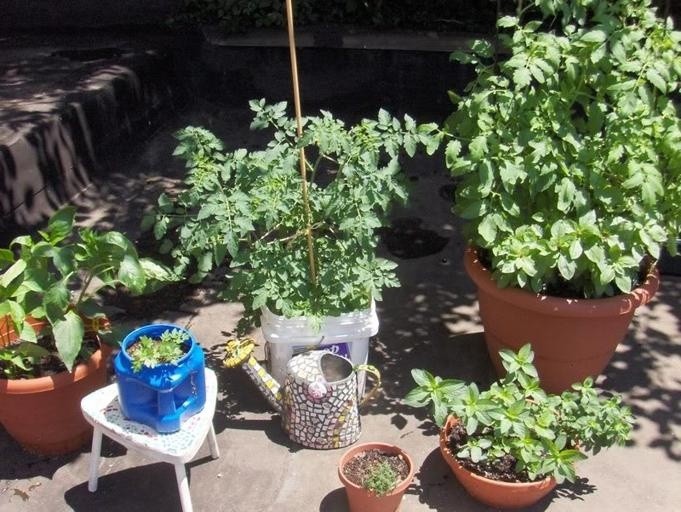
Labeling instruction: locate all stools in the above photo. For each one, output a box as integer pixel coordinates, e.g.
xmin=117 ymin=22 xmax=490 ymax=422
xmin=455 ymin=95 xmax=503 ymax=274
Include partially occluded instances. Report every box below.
xmin=80 ymin=365 xmax=220 ymax=512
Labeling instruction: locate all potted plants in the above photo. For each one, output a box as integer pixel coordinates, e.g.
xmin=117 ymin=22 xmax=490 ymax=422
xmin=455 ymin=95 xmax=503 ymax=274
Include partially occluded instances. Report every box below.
xmin=337 ymin=442 xmax=412 ymax=512
xmin=139 ymin=96 xmax=416 ymax=406
xmin=114 ymin=324 xmax=206 ymax=433
xmin=0 ymin=205 xmax=146 ymax=457
xmin=416 ymin=0 xmax=681 ymax=400
xmin=409 ymin=343 xmax=637 ymax=511
